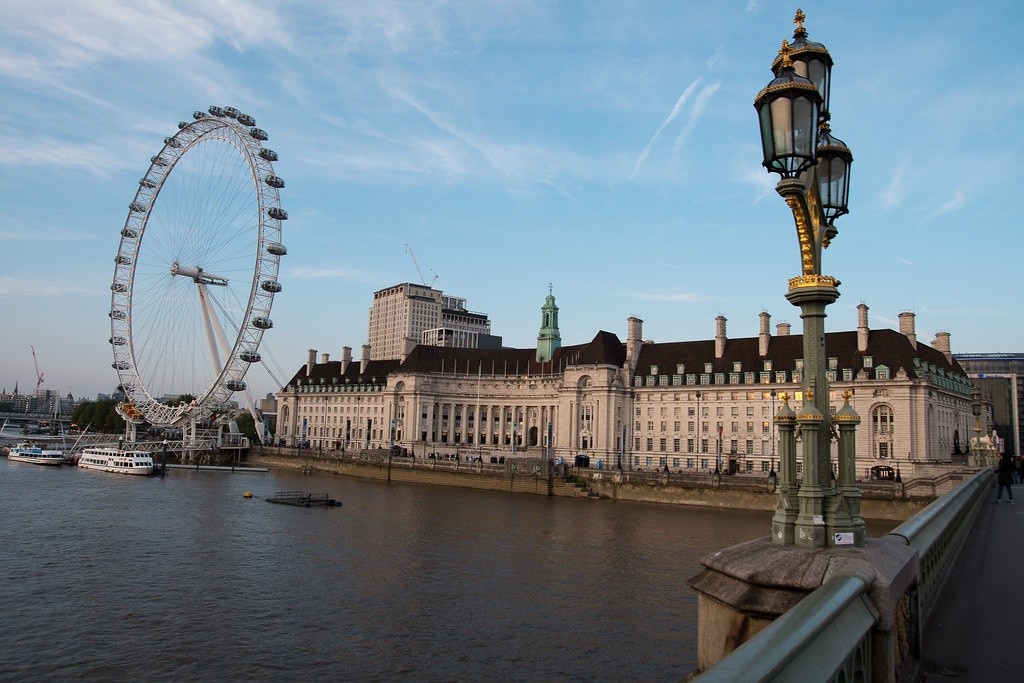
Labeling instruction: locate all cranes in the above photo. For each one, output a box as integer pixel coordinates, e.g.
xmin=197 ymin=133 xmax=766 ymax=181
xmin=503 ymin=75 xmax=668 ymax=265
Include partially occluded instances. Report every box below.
xmin=30 ymin=344 xmax=45 ymax=388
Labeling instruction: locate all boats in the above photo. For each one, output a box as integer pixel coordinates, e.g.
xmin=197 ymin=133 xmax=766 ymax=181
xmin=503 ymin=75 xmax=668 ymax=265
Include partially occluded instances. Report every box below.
xmin=69 ymin=423 xmax=81 ymax=435
xmin=8 ymin=444 xmax=65 ymax=466
xmin=268 ymin=491 xmax=342 ymax=509
xmin=78 ymin=447 xmax=153 ymax=476
xmin=21 ymin=420 xmax=57 ymax=435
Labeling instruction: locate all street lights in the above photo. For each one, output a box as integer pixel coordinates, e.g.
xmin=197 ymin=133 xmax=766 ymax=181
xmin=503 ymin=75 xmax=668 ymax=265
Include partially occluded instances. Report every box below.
xmin=971 ymin=385 xmax=982 ymax=468
